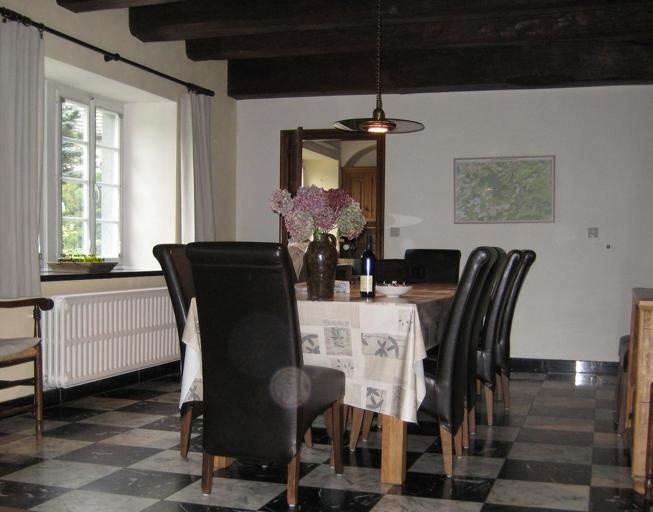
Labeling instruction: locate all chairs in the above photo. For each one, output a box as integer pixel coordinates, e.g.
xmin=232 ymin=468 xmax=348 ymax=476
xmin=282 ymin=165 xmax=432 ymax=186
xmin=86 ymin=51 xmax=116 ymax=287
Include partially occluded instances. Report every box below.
xmin=349 ymin=246 xmax=498 ymax=477
xmin=404 ymin=249 xmax=461 ymax=284
xmin=0 ymin=297 xmax=54 ymax=421
xmin=378 ymin=258 xmax=404 ymax=282
xmin=477 ymin=249 xmax=525 ymax=427
xmin=152 ymin=243 xmax=202 ymax=457
xmin=378 ymin=247 xmax=507 ymax=449
xmin=494 ymin=251 xmax=536 ymax=410
xmin=188 ymin=239 xmax=346 ymax=502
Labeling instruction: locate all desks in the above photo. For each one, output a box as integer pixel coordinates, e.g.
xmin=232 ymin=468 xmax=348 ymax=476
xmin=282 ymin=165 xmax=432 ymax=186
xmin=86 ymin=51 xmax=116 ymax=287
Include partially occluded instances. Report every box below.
xmin=617 ymin=287 xmax=653 ymax=494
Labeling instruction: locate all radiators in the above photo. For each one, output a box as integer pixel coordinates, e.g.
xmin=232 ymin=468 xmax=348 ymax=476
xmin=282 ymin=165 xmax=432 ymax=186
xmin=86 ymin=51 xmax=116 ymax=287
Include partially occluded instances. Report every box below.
xmin=34 ymin=286 xmax=178 ymax=390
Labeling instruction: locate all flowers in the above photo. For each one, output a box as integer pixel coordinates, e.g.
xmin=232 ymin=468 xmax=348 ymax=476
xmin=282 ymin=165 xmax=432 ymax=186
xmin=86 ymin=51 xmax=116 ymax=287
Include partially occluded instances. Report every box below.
xmin=270 ymin=185 xmax=367 ymax=241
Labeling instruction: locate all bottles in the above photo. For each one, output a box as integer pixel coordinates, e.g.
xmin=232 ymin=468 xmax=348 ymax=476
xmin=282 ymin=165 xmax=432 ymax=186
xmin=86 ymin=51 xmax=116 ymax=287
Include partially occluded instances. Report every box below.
xmin=359 ymin=233 xmax=380 ymax=296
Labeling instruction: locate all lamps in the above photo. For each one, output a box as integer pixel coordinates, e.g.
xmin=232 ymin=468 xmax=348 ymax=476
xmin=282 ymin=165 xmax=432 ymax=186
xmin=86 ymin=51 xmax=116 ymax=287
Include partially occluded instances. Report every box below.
xmin=333 ymin=0 xmax=425 ymax=134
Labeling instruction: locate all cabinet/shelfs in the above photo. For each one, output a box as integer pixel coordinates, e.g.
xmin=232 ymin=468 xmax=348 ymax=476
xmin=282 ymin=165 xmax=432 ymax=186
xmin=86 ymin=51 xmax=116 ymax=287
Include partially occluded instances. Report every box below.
xmin=340 ymin=167 xmax=377 ymax=224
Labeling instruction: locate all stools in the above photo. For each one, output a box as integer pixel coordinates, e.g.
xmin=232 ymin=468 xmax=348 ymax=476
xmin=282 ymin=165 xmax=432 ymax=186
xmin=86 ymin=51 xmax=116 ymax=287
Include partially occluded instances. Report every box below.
xmin=612 ymin=335 xmax=630 ymax=431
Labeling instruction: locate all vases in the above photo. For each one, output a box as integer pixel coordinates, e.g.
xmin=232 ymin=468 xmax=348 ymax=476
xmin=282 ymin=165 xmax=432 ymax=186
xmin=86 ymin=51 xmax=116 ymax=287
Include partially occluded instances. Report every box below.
xmin=304 ymin=232 xmax=338 ymax=299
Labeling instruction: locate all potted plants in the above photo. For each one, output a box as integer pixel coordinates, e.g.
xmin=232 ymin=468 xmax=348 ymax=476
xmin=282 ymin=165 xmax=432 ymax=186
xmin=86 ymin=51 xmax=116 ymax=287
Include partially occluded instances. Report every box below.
xmin=46 ymin=251 xmax=119 ymax=274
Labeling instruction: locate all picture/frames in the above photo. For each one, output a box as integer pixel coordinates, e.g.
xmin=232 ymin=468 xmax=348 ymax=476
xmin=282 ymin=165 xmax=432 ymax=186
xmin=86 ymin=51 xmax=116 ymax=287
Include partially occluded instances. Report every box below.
xmin=453 ymin=155 xmax=555 ymax=224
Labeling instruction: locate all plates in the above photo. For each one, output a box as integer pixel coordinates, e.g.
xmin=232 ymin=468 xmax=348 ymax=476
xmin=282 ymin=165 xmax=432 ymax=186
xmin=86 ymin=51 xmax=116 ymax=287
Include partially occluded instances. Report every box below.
xmin=373 ymin=280 xmax=413 ymax=299
